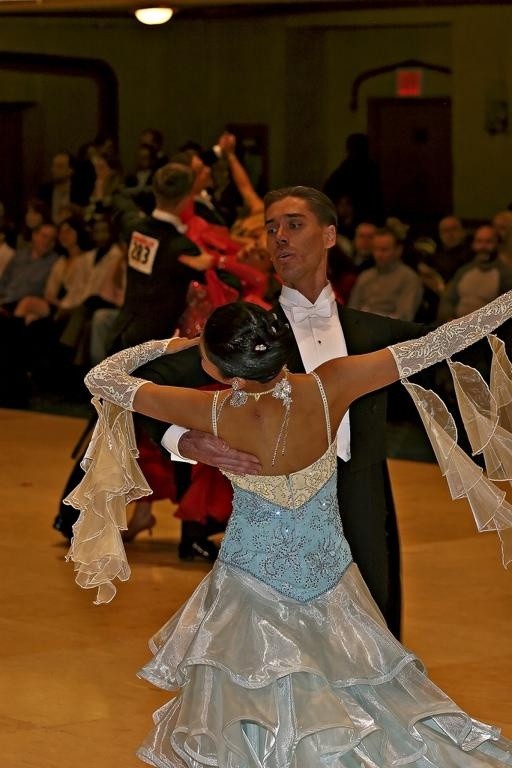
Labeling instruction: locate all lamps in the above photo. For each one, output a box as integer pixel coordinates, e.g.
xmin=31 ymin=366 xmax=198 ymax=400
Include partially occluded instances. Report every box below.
xmin=129 ymin=0 xmax=173 ymax=26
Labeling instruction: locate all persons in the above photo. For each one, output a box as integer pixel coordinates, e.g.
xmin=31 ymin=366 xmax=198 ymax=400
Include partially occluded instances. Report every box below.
xmin=131 ymin=186 xmax=512 ymax=647
xmin=62 ymin=288 xmax=511 ymax=768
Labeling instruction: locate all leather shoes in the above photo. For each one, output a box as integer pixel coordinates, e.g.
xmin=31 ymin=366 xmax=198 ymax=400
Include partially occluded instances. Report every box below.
xmin=178 ymin=540 xmax=217 ymax=563
xmin=52 ymin=515 xmax=72 ymax=540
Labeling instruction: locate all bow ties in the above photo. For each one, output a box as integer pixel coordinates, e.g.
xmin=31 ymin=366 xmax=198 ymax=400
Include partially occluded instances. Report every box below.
xmin=289 ymin=304 xmax=334 ymax=325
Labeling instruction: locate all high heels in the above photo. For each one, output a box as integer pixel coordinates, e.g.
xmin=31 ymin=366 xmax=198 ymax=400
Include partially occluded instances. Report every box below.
xmin=122 ymin=513 xmax=156 ymax=542
